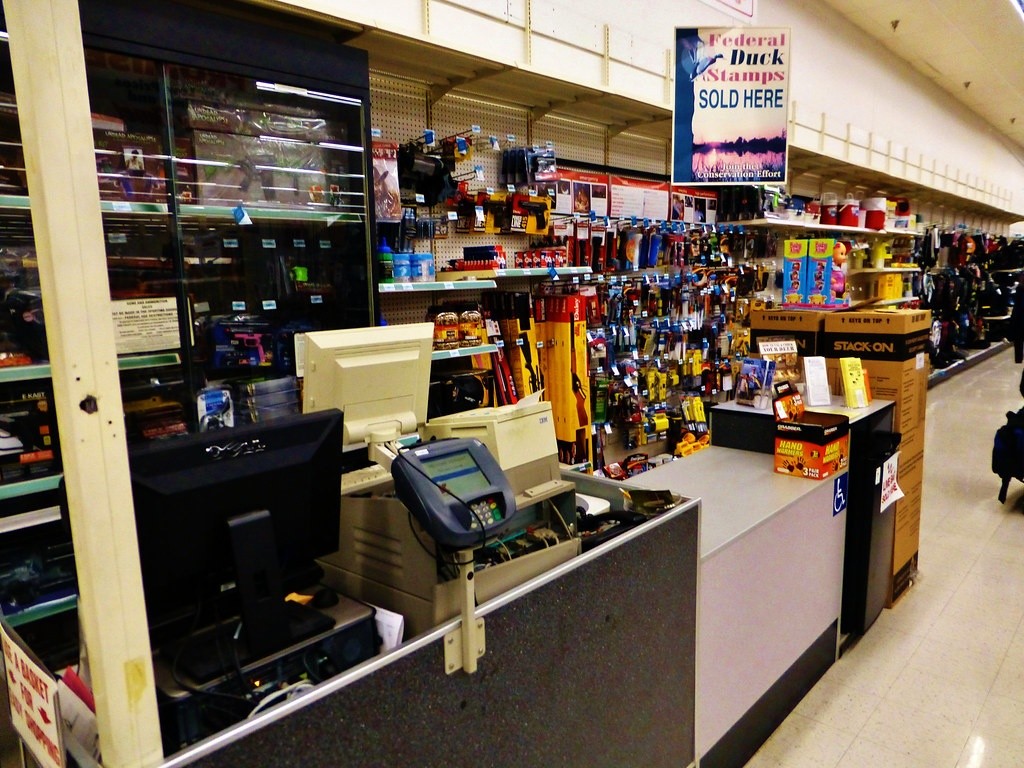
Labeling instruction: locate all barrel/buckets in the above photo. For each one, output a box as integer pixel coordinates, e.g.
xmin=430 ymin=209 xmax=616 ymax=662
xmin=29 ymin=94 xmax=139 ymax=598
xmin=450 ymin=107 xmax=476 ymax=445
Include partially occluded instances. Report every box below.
xmin=246 ymin=377 xmax=299 ymax=423
xmin=865 ymin=210 xmax=886 ymax=230
xmin=821 ymin=200 xmax=838 ymax=225
xmin=838 ymin=200 xmax=859 ymax=226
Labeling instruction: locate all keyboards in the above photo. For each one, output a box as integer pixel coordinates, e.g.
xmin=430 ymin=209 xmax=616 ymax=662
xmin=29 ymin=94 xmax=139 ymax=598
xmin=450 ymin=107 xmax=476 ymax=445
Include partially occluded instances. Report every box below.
xmin=340 ymin=463 xmax=394 ymax=495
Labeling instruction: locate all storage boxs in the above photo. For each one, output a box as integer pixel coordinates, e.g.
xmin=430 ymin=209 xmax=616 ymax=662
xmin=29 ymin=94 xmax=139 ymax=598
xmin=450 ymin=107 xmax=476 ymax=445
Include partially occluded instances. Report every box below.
xmin=749 ymin=308 xmax=832 ymax=366
xmin=824 ymin=308 xmax=932 ymax=609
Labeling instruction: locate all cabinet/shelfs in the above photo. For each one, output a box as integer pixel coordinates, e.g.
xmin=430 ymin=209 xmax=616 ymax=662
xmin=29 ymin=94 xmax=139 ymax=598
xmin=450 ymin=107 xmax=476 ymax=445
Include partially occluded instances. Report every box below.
xmin=717 ymin=218 xmax=923 ymax=305
xmin=0 ymin=31 xmax=378 ymax=629
xmin=378 ymin=266 xmax=593 ymax=362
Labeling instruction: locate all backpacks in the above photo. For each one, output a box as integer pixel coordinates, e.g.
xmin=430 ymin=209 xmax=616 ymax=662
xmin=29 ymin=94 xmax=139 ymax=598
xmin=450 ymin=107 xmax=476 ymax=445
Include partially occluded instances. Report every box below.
xmin=992 ymin=406 xmax=1024 ymax=504
xmin=929 ymin=316 xmax=942 ymax=347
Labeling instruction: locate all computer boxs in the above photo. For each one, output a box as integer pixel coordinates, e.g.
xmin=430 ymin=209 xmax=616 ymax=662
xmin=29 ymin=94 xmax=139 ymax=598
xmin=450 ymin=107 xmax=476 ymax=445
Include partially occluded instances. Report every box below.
xmin=148 ymin=580 xmax=384 ymax=759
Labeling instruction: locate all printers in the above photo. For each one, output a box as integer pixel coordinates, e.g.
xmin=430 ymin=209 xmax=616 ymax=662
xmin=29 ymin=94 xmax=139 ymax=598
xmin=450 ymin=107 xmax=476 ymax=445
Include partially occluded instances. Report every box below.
xmin=424 ymin=399 xmax=562 ymax=499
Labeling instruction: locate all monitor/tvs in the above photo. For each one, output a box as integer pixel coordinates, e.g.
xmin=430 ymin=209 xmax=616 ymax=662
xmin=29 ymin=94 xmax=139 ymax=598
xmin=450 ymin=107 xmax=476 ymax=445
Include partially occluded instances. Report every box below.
xmin=304 ymin=321 xmax=434 ymax=452
xmin=59 ymin=408 xmax=345 ymax=686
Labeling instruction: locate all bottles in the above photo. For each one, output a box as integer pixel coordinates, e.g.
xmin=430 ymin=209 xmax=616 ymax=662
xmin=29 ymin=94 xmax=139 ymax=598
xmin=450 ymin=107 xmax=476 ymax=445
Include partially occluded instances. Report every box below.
xmin=377 ymin=237 xmax=393 ymax=283
xmin=758 ymin=260 xmax=784 ymax=289
